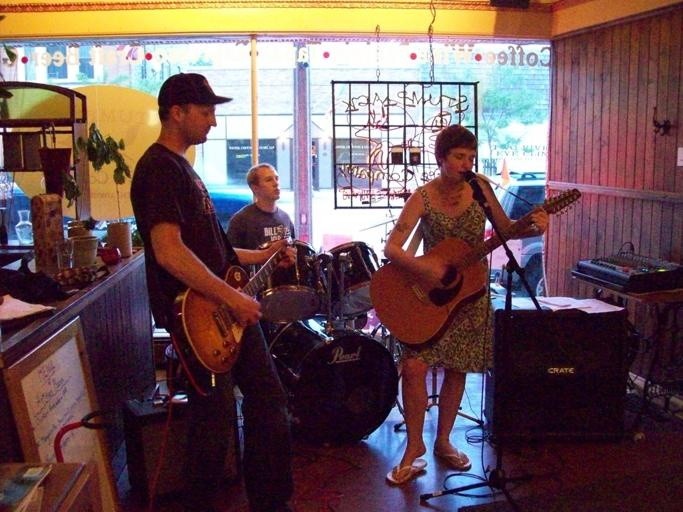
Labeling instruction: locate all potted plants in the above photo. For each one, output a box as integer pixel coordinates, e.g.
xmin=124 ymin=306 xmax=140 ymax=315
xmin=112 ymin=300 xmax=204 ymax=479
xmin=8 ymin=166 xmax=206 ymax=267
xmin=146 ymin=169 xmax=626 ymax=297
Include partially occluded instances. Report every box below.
xmin=72 ymin=123 xmax=136 ymax=258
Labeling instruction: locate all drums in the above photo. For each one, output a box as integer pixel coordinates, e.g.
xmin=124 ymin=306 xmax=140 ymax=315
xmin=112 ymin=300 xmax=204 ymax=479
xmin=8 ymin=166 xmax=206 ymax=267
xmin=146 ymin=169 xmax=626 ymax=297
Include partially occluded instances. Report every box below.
xmin=250 ymin=239 xmax=324 ymax=324
xmin=317 ymin=242 xmax=377 ymax=319
xmin=270 ymin=318 xmax=398 ymax=441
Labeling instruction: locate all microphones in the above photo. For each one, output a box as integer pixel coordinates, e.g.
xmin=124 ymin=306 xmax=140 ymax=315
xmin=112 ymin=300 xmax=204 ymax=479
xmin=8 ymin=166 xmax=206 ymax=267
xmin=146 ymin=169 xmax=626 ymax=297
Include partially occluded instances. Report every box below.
xmin=462 ymin=169 xmax=492 ymax=214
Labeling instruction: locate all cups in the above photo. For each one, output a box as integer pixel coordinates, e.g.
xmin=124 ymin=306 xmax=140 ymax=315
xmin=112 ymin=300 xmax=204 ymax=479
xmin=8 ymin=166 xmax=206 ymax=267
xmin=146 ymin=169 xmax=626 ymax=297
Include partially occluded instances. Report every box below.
xmin=55 ymin=240 xmax=74 ymax=269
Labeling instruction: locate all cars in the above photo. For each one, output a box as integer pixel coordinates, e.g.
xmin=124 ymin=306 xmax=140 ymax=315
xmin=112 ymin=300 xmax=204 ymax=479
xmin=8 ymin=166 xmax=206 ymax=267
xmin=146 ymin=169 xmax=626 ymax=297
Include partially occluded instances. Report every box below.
xmin=484 ymin=173 xmax=545 ymax=297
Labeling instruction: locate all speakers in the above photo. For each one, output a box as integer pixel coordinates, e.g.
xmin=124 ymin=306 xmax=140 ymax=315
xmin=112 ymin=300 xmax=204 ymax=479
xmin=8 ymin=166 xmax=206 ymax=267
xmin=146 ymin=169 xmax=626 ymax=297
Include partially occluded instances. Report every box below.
xmin=123 ymin=396 xmax=242 ymax=496
xmin=484 ymin=298 xmax=630 ymax=446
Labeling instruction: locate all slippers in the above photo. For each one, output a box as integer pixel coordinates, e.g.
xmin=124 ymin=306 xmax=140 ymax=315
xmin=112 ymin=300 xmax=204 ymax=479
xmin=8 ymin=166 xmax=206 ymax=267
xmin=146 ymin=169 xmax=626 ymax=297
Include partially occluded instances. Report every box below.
xmin=386 ymin=458 xmax=426 ymax=484
xmin=433 ymin=448 xmax=471 ymax=470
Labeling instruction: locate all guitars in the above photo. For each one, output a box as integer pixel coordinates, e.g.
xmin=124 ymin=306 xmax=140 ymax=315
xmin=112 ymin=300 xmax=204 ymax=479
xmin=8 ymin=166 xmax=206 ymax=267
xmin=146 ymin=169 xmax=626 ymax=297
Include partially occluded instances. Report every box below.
xmin=369 ymin=188 xmax=581 ymax=351
xmin=170 ymin=225 xmax=292 ymax=376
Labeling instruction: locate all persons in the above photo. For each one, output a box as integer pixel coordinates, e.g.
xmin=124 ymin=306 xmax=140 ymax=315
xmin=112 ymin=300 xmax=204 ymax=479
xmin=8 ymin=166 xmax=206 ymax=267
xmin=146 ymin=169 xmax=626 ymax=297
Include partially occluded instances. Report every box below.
xmin=386 ymin=124 xmax=552 ymax=484
xmin=130 ymin=73 xmax=292 ymax=511
xmin=225 ymin=163 xmax=295 ymax=287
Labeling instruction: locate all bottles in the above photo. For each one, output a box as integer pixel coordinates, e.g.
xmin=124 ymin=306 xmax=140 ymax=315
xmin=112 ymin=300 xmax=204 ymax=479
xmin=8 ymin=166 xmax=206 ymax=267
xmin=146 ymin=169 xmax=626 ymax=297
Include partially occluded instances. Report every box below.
xmin=0 ymin=207 xmax=33 ymax=245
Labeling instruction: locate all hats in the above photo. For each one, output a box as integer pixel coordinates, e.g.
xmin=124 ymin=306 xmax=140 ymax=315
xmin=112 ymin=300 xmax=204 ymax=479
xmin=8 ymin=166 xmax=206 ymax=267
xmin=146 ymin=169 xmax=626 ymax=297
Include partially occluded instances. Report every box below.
xmin=158 ymin=73 xmax=232 ymax=107
